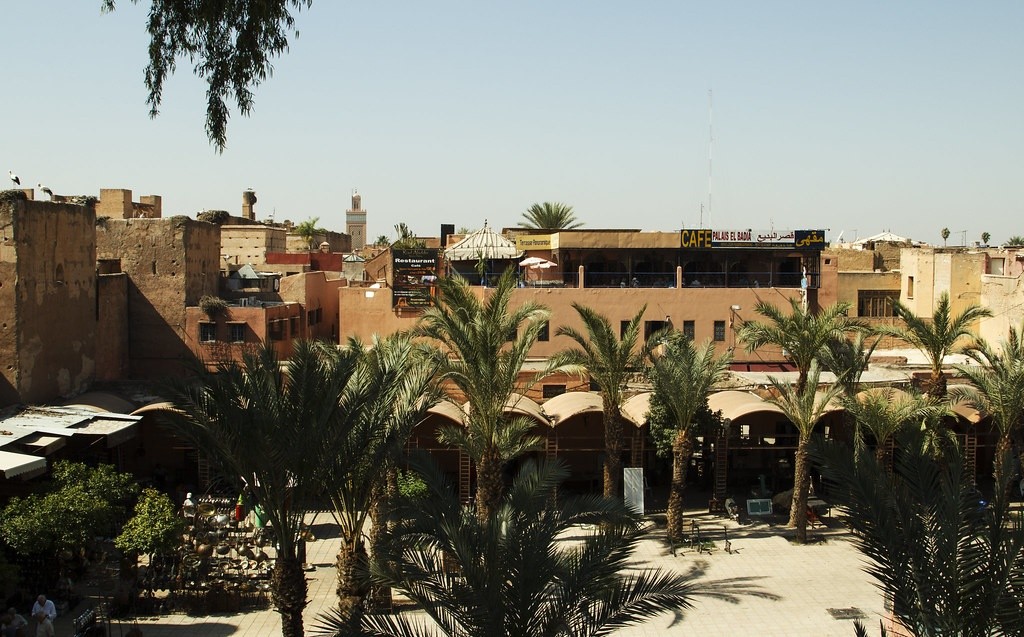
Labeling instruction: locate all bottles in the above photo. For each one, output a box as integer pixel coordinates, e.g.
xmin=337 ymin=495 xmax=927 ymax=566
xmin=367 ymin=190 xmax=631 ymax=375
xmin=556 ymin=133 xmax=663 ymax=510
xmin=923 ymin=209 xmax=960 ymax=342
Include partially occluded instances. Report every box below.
xmin=234 ymin=494 xmax=245 ymax=521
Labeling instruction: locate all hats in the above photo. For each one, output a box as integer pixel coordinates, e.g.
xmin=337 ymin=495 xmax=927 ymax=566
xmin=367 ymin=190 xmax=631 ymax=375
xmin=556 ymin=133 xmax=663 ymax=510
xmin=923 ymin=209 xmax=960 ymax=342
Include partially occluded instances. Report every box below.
xmin=186 ymin=493 xmax=191 ymax=498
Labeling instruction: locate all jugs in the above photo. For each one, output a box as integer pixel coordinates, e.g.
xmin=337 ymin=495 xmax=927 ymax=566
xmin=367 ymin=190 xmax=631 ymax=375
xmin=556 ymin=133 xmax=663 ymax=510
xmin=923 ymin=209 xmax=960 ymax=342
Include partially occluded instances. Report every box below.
xmin=196 ymin=513 xmax=268 ymax=557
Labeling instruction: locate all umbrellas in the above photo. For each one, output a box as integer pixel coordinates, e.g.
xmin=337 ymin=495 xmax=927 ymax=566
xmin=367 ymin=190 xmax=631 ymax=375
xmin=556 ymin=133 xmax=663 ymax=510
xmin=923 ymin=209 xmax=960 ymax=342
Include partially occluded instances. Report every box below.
xmin=518 ymin=257 xmax=558 ymax=288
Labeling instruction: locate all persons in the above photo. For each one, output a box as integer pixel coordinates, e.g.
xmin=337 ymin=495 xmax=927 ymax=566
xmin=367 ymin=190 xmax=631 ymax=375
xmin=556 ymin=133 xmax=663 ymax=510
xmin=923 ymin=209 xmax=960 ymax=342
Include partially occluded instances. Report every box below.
xmin=0 ymin=607 xmax=28 ymax=637
xmin=183 ymin=492 xmax=197 ymax=522
xmin=32 ymin=594 xmax=57 ymax=637
xmin=652 ymin=277 xmax=675 ymax=287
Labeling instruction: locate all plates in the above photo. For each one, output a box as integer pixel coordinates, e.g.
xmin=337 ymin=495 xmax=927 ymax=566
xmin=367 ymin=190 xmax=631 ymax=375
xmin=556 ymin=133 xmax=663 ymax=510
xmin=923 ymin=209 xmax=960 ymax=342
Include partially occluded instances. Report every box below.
xmin=198 ymin=503 xmax=216 ymax=515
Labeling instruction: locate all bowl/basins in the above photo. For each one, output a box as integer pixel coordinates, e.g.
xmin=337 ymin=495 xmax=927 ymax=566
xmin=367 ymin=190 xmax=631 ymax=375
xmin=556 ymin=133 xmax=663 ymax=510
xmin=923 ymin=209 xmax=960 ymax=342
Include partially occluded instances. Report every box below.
xmin=209 ymin=558 xmax=269 ymax=571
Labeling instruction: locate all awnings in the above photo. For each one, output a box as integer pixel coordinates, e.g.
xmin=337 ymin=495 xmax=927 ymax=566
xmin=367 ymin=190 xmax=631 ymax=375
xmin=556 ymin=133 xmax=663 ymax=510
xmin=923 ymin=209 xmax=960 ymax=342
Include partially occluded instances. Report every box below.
xmin=462 ymin=394 xmax=550 ymax=426
xmin=621 ymin=391 xmax=655 ymax=427
xmin=428 ymin=401 xmax=463 ymax=424
xmin=707 ymin=391 xmax=789 ymax=420
xmin=922 ymin=384 xmax=989 ymax=424
xmin=856 ymin=388 xmax=912 ymax=411
xmin=777 ymin=390 xmax=849 ymax=422
xmin=542 ymin=391 xmax=635 ymax=425
